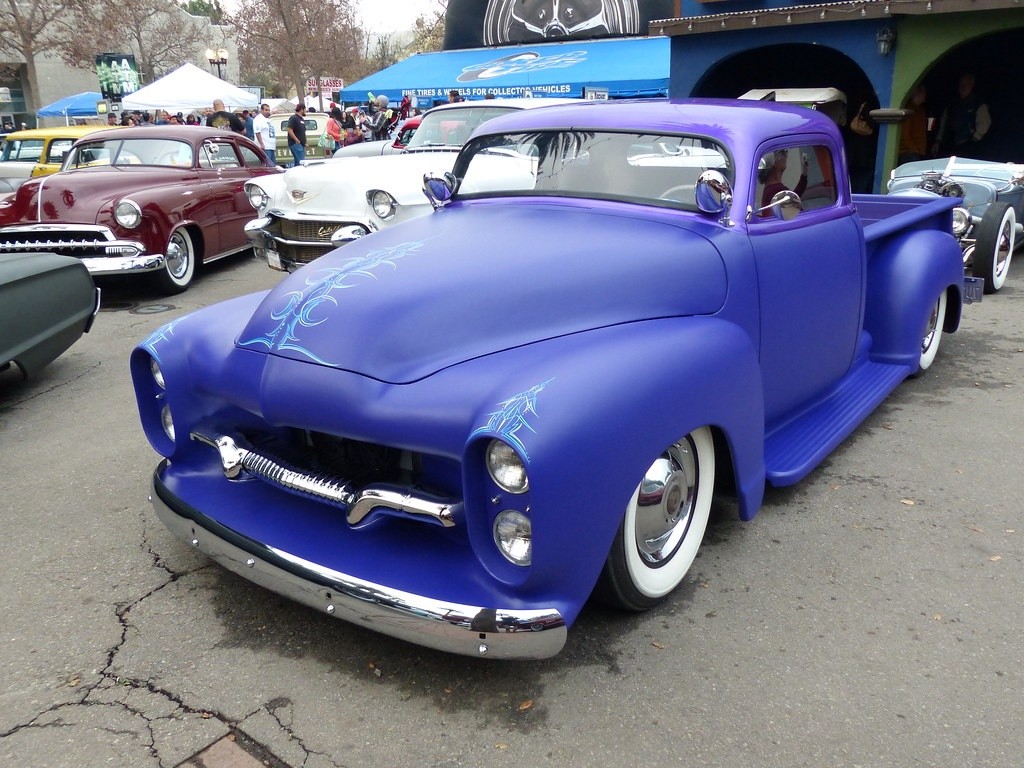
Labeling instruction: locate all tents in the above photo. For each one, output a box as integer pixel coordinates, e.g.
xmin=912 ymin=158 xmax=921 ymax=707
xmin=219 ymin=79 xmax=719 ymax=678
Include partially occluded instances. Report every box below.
xmin=291 ymin=95 xmax=343 ymax=112
xmin=262 ymin=98 xmax=296 ymax=113
xmin=36 ymin=92 xmax=112 ymax=129
xmin=122 ymin=63 xmax=259 ymax=110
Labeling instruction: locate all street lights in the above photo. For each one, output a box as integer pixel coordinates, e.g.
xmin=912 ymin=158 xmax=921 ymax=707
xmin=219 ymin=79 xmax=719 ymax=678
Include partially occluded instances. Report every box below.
xmin=205 ymin=48 xmax=229 ymax=79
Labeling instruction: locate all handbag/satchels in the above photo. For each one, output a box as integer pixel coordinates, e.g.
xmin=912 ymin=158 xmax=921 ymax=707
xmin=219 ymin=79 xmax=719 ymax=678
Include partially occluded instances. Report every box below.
xmin=318 ymin=130 xmax=335 ymax=150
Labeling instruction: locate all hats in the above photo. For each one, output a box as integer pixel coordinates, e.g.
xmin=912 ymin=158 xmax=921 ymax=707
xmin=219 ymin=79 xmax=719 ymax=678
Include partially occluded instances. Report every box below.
xmin=377 ymin=94 xmax=389 ymax=109
xmin=330 ymin=103 xmax=335 ymax=108
xmin=296 ymin=104 xmax=305 ymax=111
xmin=178 ymin=112 xmax=183 ymax=117
xmin=453 ymin=96 xmax=465 ymax=102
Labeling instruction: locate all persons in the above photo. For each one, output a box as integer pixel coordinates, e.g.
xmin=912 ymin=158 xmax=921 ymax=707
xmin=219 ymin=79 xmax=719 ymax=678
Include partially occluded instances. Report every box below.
xmin=200 ymin=108 xmax=212 ymax=126
xmin=107 ymin=110 xmax=152 ymax=126
xmin=759 ymin=149 xmax=809 ymax=215
xmin=288 ymin=104 xmax=308 ymax=164
xmin=898 ymin=73 xmax=990 ymax=165
xmin=448 ymin=91 xmax=465 ymax=103
xmin=254 ymin=103 xmax=276 ymax=160
xmin=242 ymin=110 xmax=254 ymax=140
xmin=252 ymin=109 xmax=258 ymax=117
xmin=327 ymin=95 xmax=391 ymax=154
xmin=157 ymin=111 xmax=199 ymax=126
xmin=206 ymin=99 xmax=245 ymax=134
xmin=0 ymin=123 xmax=15 ymax=151
xmin=485 ymin=93 xmax=494 ymax=99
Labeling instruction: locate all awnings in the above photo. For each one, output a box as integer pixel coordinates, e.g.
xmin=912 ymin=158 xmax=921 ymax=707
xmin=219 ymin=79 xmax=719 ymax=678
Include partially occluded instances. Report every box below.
xmin=341 ymin=36 xmax=670 ymax=108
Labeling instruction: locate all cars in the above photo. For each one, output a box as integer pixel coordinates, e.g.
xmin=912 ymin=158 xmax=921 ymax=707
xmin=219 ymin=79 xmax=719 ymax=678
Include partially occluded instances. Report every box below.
xmin=119 ymin=95 xmax=989 ymax=666
xmin=883 ymin=154 xmax=1024 ymax=295
xmin=0 ymin=86 xmax=851 ymax=387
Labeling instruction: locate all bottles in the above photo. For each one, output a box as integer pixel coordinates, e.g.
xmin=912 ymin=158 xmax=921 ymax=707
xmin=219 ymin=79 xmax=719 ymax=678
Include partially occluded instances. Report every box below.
xmin=340 ymin=129 xmax=344 ymax=140
